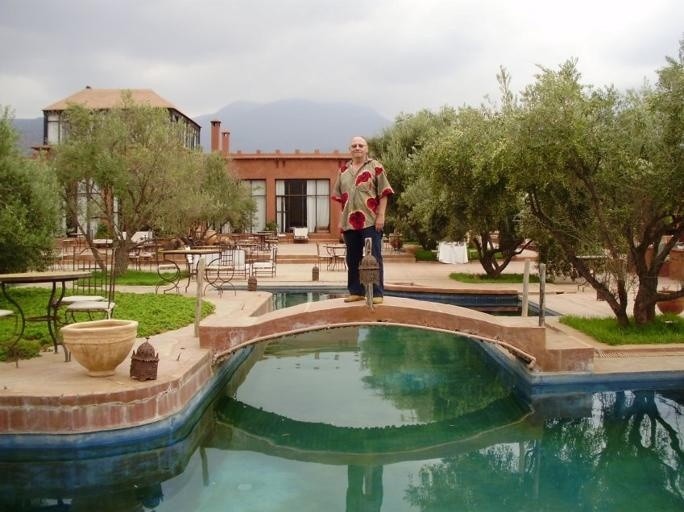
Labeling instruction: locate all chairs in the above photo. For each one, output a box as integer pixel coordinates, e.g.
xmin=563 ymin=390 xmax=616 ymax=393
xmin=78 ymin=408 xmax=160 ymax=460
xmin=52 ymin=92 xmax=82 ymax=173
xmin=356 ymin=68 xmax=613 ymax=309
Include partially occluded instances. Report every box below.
xmin=316 ymin=239 xmax=347 ymax=273
xmin=155 ymin=236 xmax=278 ymax=299
xmin=56 ymin=244 xmax=122 ymax=362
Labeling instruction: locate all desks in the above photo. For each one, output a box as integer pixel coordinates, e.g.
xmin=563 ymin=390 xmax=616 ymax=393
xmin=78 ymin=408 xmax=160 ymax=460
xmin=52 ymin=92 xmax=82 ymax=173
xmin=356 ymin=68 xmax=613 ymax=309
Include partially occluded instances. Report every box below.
xmin=1 ymin=271 xmax=93 ymax=368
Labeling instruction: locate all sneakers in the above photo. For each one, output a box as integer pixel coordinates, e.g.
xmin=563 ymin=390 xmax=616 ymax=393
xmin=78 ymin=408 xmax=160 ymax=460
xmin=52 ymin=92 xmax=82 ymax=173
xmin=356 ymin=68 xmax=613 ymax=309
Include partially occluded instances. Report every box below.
xmin=373 ymin=297 xmax=382 ymax=304
xmin=344 ymin=295 xmax=364 ymax=302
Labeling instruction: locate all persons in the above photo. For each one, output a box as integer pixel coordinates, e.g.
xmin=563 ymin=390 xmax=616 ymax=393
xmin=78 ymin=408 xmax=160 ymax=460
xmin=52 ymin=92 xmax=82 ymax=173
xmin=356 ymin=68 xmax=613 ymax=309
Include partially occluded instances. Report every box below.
xmin=329 ymin=136 xmax=395 ymax=305
